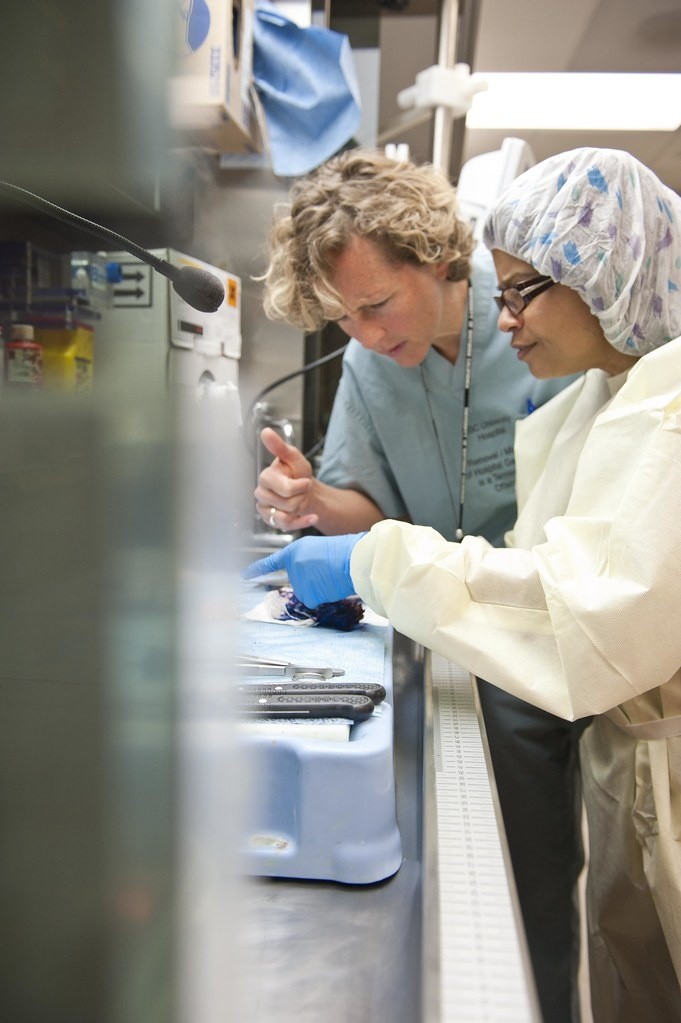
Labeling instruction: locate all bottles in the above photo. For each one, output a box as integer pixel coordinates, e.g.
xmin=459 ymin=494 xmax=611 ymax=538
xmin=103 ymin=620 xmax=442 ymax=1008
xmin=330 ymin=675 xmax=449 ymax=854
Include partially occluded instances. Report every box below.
xmin=4 ymin=325 xmax=43 ymax=387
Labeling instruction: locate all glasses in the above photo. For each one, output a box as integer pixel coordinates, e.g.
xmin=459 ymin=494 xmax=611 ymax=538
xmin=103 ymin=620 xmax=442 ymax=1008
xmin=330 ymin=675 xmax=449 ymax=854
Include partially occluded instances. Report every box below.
xmin=492 ymin=275 xmax=558 ymax=316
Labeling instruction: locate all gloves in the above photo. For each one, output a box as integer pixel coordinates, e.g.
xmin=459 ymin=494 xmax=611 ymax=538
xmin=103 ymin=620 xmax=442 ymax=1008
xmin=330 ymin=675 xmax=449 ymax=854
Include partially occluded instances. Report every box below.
xmin=236 ymin=531 xmax=366 ymax=610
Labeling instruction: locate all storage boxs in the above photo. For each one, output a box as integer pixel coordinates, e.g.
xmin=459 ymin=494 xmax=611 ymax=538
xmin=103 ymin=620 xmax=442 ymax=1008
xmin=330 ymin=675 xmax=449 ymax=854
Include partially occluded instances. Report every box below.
xmin=167 ymin=0 xmax=275 ymax=175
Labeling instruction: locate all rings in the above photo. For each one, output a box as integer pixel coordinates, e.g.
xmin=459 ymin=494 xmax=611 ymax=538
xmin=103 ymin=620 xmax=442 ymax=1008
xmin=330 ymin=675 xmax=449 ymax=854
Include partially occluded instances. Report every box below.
xmin=267 ymin=507 xmax=278 ymax=528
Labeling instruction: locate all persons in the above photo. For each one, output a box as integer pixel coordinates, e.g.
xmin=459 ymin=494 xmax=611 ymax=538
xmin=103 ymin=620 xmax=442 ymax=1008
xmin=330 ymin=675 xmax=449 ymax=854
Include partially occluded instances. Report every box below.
xmin=251 ymin=153 xmax=587 ymax=1023
xmin=239 ymin=147 xmax=681 ymax=1023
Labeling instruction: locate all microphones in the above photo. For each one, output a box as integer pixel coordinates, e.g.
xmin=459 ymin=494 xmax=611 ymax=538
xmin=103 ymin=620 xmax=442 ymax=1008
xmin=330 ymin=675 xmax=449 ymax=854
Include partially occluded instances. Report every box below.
xmin=0 ymin=179 xmax=225 ymax=312
